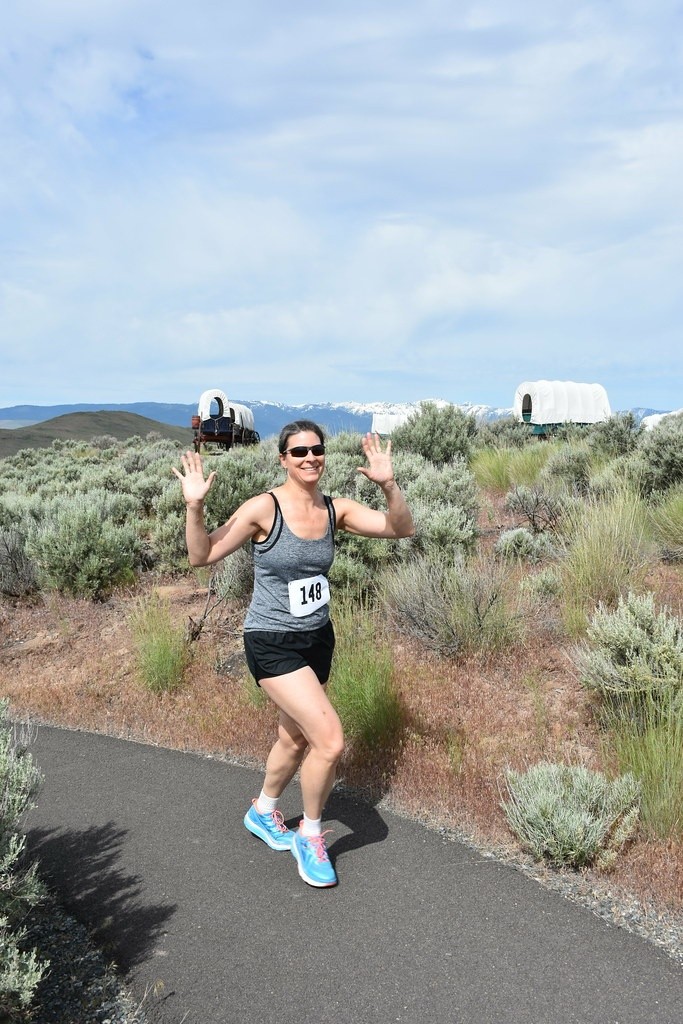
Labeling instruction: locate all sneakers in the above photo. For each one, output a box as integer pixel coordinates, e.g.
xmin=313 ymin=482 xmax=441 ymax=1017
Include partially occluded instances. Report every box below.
xmin=291 ymin=820 xmax=337 ymax=887
xmin=244 ymin=798 xmax=296 ymax=851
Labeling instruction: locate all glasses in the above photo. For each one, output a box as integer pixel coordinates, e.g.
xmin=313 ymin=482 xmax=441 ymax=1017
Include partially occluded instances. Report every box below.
xmin=283 ymin=445 xmax=326 ymax=457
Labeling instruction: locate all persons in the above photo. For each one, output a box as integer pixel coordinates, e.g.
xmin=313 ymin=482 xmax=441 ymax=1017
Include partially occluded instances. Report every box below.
xmin=172 ymin=421 xmax=414 ymax=886
